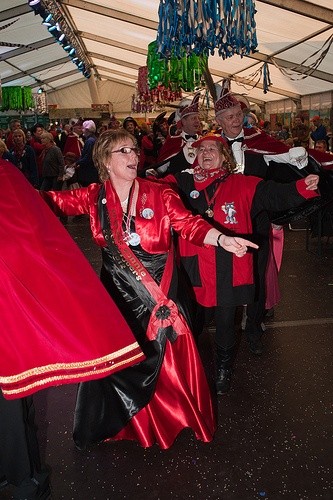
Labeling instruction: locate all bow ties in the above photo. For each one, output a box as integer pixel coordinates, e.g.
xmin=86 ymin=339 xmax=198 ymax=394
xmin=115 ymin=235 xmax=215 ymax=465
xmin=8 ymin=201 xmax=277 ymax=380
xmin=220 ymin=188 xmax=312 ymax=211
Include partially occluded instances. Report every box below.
xmin=229 ymin=137 xmax=244 ymax=145
xmin=185 ymin=134 xmax=197 ymax=140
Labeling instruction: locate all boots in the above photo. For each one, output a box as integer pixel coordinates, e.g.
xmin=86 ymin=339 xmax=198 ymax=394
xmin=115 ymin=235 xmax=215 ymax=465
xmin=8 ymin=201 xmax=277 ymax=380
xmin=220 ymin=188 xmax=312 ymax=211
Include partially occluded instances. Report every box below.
xmin=214 ymin=342 xmax=236 ymax=395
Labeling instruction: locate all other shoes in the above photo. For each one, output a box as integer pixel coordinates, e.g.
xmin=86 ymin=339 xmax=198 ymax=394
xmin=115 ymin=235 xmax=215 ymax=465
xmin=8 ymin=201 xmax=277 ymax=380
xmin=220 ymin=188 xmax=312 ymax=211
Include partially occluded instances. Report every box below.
xmin=246 ymin=331 xmax=265 ymax=354
xmin=264 ymin=312 xmax=273 ymax=327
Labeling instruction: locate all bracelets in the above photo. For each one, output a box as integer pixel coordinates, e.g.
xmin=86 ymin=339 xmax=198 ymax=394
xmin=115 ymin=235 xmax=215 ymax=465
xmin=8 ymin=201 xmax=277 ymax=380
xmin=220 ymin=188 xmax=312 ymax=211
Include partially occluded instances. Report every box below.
xmin=217 ymin=233 xmax=224 ymax=248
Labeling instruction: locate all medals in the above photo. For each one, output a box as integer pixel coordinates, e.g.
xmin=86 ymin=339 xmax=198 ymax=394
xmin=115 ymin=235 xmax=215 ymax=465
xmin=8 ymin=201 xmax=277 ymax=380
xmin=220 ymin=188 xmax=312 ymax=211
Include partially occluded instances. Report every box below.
xmin=205 ymin=208 xmax=214 ymax=217
xmin=128 ymin=232 xmax=141 ymax=246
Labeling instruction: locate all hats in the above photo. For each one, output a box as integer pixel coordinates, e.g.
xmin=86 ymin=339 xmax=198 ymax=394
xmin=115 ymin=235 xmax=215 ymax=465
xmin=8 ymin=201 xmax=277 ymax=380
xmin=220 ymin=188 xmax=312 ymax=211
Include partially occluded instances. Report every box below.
xmin=214 ymin=78 xmax=250 ymax=116
xmin=191 ymin=132 xmax=228 ymax=148
xmin=174 ymin=92 xmax=201 ymax=122
xmin=123 ymin=117 xmax=137 ymax=129
xmin=310 ymin=116 xmax=320 ymax=122
xmin=83 ymin=120 xmax=96 ymax=133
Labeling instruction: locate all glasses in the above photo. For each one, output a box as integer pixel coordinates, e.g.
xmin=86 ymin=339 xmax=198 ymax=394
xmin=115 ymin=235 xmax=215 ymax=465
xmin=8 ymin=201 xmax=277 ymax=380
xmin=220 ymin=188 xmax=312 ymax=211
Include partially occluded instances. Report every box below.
xmin=109 ymin=147 xmax=141 ymax=155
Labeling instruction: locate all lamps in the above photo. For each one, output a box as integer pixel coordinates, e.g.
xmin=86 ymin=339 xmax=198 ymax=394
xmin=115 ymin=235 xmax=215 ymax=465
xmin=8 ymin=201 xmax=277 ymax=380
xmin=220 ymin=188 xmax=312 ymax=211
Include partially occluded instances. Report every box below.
xmin=27 ymin=0 xmax=91 ymax=79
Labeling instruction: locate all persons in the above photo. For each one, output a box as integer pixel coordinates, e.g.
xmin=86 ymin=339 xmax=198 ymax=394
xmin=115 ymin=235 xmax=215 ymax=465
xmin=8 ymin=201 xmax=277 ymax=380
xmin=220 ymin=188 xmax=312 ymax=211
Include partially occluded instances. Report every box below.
xmin=35 ymin=129 xmax=260 ymax=450
xmin=0 ymin=92 xmax=333 ymax=357
xmin=145 ymin=136 xmax=321 ymax=394
xmin=0 ymin=157 xmax=147 ymax=500
